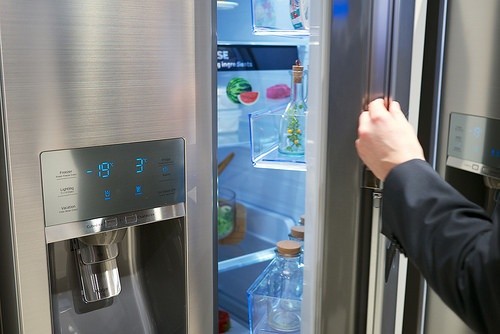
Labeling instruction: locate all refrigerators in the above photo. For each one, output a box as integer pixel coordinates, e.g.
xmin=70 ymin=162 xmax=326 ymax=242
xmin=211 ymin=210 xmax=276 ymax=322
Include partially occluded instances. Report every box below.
xmin=0 ymin=0 xmax=499 ymax=334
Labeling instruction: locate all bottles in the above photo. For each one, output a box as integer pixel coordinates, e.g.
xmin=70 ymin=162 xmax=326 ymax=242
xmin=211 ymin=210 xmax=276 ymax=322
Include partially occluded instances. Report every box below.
xmin=278 ymin=60 xmax=306 ymax=155
xmin=269 ymin=214 xmax=305 ymax=333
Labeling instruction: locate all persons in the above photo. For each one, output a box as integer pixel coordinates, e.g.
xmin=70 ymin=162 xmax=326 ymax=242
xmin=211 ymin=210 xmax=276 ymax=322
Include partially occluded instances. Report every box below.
xmin=355 ymin=92 xmax=500 ymax=332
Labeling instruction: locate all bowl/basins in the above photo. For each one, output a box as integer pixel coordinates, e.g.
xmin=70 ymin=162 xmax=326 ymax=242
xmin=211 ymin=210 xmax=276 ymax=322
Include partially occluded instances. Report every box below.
xmin=216 ymin=188 xmax=235 ymax=241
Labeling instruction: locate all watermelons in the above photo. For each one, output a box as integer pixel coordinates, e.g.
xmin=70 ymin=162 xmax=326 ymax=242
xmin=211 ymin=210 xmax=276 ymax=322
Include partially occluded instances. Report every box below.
xmin=226 ymin=76 xmax=259 ymax=105
